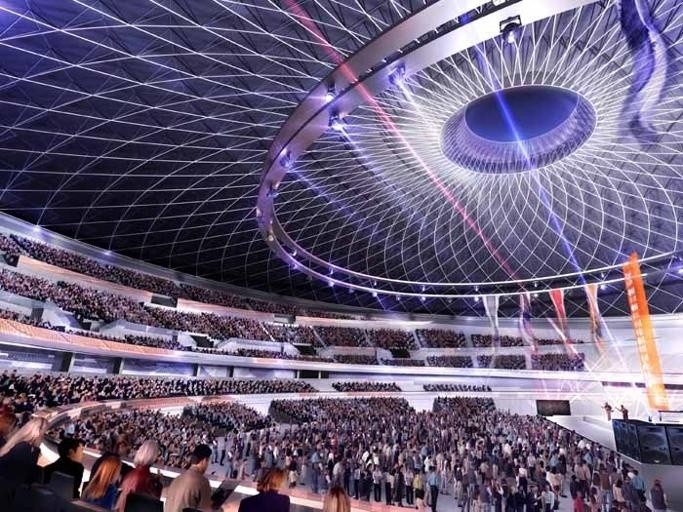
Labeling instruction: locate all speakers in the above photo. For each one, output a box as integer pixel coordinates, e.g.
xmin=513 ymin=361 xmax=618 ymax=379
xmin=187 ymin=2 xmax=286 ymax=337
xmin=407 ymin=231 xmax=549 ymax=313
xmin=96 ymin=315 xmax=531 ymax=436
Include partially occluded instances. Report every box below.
xmin=612 ymin=419 xmax=683 ymax=466
xmin=536 ymin=400 xmax=570 ymax=416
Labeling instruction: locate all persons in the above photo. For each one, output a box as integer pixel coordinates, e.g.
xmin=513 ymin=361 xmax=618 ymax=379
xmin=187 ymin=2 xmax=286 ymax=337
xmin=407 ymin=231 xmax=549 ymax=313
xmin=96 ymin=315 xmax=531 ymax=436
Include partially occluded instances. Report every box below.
xmin=0 ymin=233 xmax=668 ymax=511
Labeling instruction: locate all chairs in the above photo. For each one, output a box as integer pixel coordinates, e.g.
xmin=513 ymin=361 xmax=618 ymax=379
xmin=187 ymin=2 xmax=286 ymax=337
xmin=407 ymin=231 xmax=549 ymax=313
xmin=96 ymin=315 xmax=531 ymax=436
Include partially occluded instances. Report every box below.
xmin=1 ymin=463 xmax=204 ymax=512
